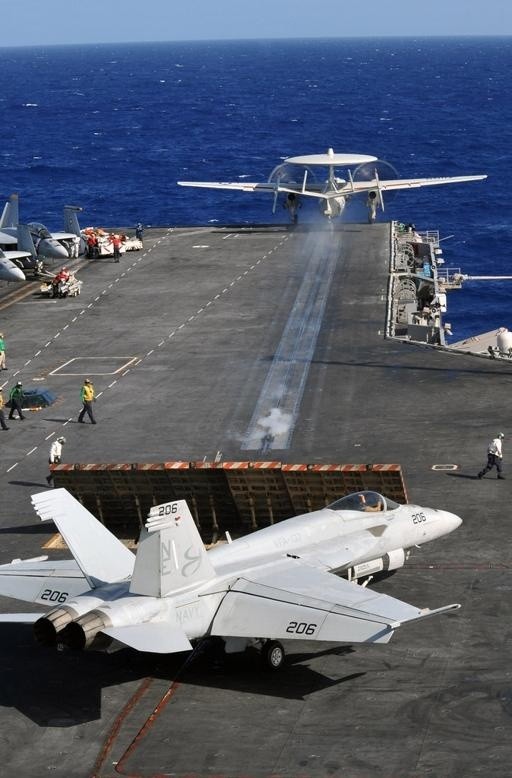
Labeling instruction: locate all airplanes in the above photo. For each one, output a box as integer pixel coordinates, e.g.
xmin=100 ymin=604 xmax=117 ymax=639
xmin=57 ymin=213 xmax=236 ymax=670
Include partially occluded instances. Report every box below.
xmin=174 ymin=149 xmax=488 ymax=224
xmin=0 ymin=192 xmax=78 ymax=283
xmin=0 ymin=490 xmax=463 ymax=673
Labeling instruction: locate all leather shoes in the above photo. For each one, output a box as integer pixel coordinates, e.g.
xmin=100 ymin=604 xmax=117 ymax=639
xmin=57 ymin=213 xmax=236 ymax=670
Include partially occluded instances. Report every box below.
xmin=92 ymin=422 xmax=97 ymax=424
xmin=78 ymin=420 xmax=85 ymax=423
xmin=2 ymin=415 xmax=26 ymax=431
xmin=498 ymin=474 xmax=507 ymax=480
xmin=477 ymin=473 xmax=485 ymax=480
xmin=46 ymin=477 xmax=51 ymax=485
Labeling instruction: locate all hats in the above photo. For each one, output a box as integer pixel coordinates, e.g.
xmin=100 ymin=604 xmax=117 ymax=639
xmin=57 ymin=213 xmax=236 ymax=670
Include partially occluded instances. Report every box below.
xmin=85 ymin=379 xmax=89 ymax=383
xmin=17 ymin=382 xmax=22 ymax=386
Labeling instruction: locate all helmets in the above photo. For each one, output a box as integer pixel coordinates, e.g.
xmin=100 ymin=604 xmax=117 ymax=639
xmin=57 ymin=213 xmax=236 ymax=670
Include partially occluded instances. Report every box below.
xmin=498 ymin=432 xmax=506 ymax=440
xmin=57 ymin=436 xmax=66 ymax=444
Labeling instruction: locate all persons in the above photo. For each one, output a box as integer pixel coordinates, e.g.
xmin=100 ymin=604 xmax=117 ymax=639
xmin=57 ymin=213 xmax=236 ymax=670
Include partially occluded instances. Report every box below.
xmin=78 ymin=378 xmax=98 ymax=425
xmin=477 ymin=431 xmax=507 ymax=480
xmin=45 ymin=436 xmax=67 ymax=488
xmin=0 ymin=333 xmax=8 ymax=372
xmin=8 ymin=381 xmax=27 ymax=420
xmin=51 ymin=220 xmax=145 ymax=299
xmin=0 ymin=387 xmax=11 ymax=431
